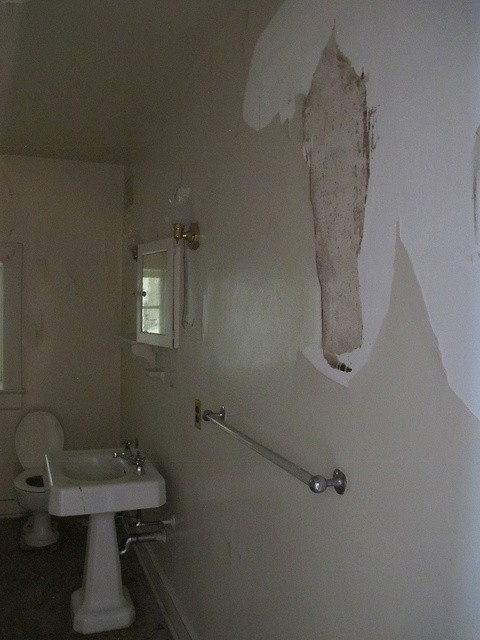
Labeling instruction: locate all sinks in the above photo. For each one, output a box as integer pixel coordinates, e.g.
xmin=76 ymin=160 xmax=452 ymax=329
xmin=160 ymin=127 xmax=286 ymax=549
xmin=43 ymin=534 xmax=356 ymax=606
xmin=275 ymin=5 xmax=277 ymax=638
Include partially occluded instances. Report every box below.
xmin=43 ymin=446 xmax=166 ymax=517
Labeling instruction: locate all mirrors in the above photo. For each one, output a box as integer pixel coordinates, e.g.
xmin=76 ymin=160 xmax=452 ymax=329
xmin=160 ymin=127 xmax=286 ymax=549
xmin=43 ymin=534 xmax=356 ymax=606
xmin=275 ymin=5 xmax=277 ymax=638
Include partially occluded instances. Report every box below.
xmin=136 ymin=235 xmax=173 ymax=349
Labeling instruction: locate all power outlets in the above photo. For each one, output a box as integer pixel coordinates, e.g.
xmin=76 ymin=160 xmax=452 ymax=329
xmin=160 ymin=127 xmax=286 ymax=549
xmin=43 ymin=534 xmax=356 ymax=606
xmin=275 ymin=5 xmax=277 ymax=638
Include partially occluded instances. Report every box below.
xmin=194 ymin=399 xmax=201 ymax=430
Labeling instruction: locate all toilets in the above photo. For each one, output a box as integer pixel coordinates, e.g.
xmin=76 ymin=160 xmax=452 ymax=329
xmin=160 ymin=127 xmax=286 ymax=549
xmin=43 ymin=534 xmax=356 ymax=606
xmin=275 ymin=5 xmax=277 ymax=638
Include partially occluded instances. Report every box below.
xmin=14 ymin=410 xmax=65 ymax=547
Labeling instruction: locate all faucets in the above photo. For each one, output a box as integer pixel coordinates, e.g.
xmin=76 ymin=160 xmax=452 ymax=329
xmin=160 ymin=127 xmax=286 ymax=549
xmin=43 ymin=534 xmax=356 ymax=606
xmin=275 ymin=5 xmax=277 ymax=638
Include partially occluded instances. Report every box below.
xmin=112 ymin=439 xmax=145 ymax=473
